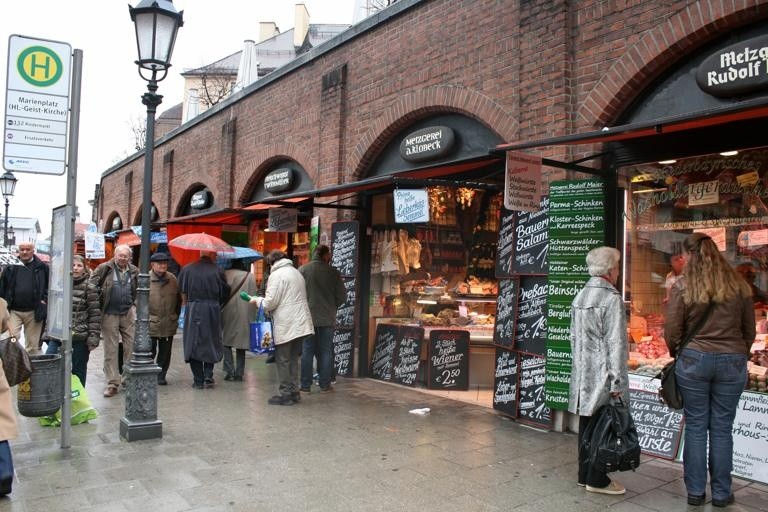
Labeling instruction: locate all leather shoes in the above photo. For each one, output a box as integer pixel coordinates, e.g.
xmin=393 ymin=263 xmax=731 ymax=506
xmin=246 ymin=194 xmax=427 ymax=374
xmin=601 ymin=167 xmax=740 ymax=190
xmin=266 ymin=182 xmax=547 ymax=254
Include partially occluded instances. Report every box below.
xmin=224 ymin=371 xmax=235 ymax=380
xmin=104 ymin=386 xmax=118 ymax=397
xmin=233 ymin=373 xmax=243 ymax=381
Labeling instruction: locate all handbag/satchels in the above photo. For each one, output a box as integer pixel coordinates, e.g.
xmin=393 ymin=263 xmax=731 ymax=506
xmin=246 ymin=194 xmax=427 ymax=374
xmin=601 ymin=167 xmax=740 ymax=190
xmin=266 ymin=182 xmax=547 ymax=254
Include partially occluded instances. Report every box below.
xmin=249 ymin=299 xmax=275 ymax=355
xmin=649 ymin=360 xmax=683 ymax=410
xmin=0 ymin=335 xmax=34 ymax=387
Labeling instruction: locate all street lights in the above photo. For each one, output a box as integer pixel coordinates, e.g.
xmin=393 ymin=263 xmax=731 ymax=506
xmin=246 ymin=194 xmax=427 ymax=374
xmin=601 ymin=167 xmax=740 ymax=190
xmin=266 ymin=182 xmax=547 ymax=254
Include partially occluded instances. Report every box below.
xmin=119 ymin=0 xmax=184 ymax=444
xmin=0 ymin=168 xmax=17 ymax=247
xmin=5 ymin=224 xmax=16 ymax=254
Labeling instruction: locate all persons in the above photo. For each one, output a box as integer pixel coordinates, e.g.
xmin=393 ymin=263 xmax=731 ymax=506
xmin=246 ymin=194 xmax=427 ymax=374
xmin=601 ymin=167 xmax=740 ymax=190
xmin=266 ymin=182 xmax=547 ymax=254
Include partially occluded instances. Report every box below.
xmin=220 ymin=257 xmax=257 ymax=381
xmin=567 ymin=247 xmax=629 ymax=495
xmin=664 ymin=232 xmax=756 ymax=507
xmin=1 ymin=299 xmax=21 ymax=499
xmin=664 ymin=243 xmax=687 ymax=300
xmin=247 ymin=245 xmax=348 ymax=405
xmin=117 ymin=252 xmax=180 ymax=386
xmin=41 ymin=255 xmax=102 ymax=388
xmin=177 ymin=250 xmax=230 ymax=388
xmin=95 ymin=245 xmax=139 ymax=397
xmin=1 ymin=240 xmax=49 ymax=355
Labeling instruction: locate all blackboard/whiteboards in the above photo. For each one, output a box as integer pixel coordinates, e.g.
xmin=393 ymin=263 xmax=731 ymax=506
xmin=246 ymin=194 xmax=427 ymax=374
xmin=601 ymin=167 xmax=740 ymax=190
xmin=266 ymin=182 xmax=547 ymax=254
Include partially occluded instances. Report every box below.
xmin=368 ymin=323 xmax=425 ymax=388
xmin=493 ymin=195 xmax=555 ymax=430
xmin=427 ymin=331 xmax=470 ymax=391
xmin=627 ymin=388 xmax=685 ymax=461
xmin=329 ymin=221 xmax=360 ymax=379
xmin=704 ymin=391 xmax=768 ymax=484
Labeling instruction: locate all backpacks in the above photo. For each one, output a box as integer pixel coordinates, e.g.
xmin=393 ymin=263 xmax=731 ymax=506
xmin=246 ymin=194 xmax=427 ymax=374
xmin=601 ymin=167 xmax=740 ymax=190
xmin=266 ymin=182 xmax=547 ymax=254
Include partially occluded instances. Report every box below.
xmin=579 ymin=393 xmax=641 ymax=474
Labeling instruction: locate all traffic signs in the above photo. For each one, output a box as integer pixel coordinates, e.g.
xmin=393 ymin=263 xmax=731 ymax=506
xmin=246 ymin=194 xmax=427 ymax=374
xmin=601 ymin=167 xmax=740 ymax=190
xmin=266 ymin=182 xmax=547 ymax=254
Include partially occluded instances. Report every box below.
xmin=3 ymin=34 xmax=72 ymax=176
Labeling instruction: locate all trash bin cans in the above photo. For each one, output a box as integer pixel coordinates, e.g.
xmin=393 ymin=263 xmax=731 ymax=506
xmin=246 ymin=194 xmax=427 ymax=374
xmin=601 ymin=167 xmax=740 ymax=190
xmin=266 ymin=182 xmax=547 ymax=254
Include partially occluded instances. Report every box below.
xmin=18 ymin=354 xmax=62 ymax=416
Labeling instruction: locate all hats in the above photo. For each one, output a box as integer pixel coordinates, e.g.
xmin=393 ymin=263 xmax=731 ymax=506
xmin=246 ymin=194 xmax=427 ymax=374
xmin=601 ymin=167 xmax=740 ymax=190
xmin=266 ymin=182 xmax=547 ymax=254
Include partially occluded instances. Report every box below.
xmin=150 ymin=252 xmax=171 ymax=262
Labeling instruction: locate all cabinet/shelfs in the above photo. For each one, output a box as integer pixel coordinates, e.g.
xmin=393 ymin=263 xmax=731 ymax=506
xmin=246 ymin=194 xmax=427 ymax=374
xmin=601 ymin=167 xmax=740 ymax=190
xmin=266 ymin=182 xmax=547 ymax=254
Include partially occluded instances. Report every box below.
xmin=396 ymin=294 xmax=497 ymax=390
xmin=415 ymin=223 xmax=499 ymax=279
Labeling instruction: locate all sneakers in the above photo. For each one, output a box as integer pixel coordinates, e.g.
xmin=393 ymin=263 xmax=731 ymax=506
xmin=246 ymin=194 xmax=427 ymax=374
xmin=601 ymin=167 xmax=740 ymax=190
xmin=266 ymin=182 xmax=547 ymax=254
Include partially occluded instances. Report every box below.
xmin=576 ymin=480 xmax=586 ymax=487
xmin=158 ymin=374 xmax=168 ymax=385
xmin=296 ymin=385 xmax=311 ymax=395
xmin=688 ymin=492 xmax=706 ymax=506
xmin=320 ymin=386 xmax=335 ymax=394
xmin=316 ymin=379 xmax=337 ymax=386
xmin=267 ymin=395 xmax=293 ymax=406
xmin=204 ymin=375 xmax=215 ymax=385
xmin=586 ymin=477 xmax=626 ymax=495
xmin=191 ymin=379 xmax=204 ymax=389
xmin=272 ymin=393 xmax=301 ymax=402
xmin=712 ymin=494 xmax=735 ymax=507
xmin=313 ymin=373 xmax=320 ymax=382
